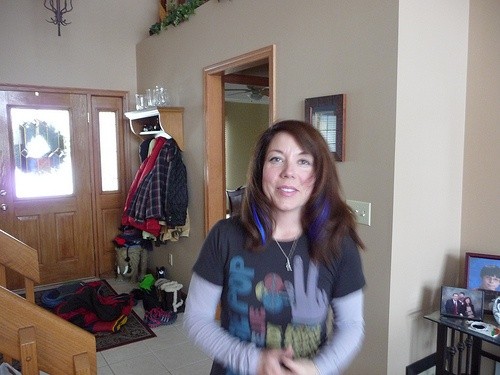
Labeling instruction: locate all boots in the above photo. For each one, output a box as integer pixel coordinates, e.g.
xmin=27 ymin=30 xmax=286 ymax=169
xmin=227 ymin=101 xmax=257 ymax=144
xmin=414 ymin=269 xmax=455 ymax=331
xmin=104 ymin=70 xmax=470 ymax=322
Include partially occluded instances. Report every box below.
xmin=122 ymin=291 xmax=137 ymax=316
xmin=165 ymin=283 xmax=184 ymax=314
xmin=161 ymin=281 xmax=177 ymax=309
xmin=155 ymin=278 xmax=169 ymax=304
xmin=89 ymin=315 xmax=128 ymax=333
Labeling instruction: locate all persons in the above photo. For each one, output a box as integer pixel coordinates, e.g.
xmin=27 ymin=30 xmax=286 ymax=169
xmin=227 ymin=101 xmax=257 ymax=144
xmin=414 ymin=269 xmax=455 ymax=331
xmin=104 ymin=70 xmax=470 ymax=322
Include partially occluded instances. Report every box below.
xmin=183 ymin=120 xmax=366 ymax=375
xmin=444 ymin=292 xmax=476 ymax=318
xmin=475 ymin=265 xmax=500 ymax=291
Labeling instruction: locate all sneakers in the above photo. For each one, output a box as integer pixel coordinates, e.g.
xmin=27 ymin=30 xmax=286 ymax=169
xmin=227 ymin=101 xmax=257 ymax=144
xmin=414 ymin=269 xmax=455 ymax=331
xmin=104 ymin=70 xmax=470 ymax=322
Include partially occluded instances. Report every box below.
xmin=144 ymin=306 xmax=178 ymax=328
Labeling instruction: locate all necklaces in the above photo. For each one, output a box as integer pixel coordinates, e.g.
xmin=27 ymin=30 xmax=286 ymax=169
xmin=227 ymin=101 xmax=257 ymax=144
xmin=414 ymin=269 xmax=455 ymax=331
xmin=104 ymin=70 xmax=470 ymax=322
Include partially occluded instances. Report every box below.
xmin=273 ymin=234 xmax=299 ymax=271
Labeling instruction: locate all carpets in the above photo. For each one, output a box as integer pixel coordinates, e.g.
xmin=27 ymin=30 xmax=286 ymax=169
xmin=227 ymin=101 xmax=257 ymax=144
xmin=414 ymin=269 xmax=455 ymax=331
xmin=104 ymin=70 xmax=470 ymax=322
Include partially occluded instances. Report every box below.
xmin=19 ymin=279 xmax=157 ymax=353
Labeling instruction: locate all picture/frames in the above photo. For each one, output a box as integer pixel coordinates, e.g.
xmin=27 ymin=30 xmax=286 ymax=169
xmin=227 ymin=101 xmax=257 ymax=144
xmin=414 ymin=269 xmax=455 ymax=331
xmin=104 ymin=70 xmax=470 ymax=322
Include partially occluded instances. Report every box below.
xmin=440 ymin=285 xmax=484 ymax=322
xmin=464 ymin=252 xmax=500 ymax=314
xmin=305 ymin=94 xmax=346 ymax=162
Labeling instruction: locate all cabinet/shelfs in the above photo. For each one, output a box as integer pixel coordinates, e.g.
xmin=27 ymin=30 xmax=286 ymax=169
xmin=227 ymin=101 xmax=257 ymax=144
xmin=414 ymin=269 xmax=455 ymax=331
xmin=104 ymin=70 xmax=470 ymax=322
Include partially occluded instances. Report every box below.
xmin=123 ymin=107 xmax=184 ymax=153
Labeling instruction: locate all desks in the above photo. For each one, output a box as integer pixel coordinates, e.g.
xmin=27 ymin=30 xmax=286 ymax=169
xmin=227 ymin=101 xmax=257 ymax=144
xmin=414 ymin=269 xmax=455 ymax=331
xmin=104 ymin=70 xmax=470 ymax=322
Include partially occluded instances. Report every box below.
xmin=424 ymin=310 xmax=500 ymax=375
